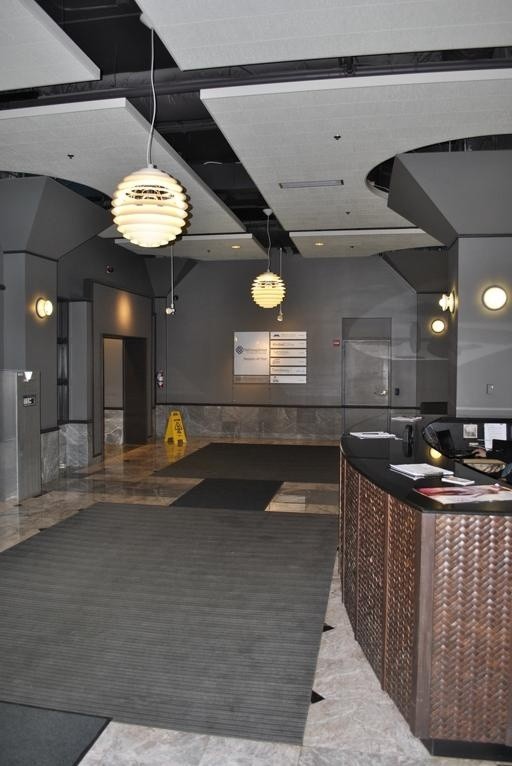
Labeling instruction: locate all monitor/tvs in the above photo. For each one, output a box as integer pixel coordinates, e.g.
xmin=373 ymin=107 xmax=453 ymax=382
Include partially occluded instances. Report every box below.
xmin=435 ymin=429 xmax=455 ymax=450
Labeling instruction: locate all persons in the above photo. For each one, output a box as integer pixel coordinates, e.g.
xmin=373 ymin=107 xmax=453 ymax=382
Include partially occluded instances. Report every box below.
xmin=471 ymin=447 xmax=512 ymax=485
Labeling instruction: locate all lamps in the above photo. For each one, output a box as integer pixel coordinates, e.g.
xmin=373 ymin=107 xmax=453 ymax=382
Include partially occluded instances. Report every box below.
xmin=432 ymin=319 xmax=445 ymax=333
xmin=250 ymin=208 xmax=286 ymax=309
xmin=111 ymin=13 xmax=188 ymax=248
xmin=166 ymin=246 xmax=176 ymax=316
xmin=277 ymin=248 xmax=284 ymax=321
xmin=439 ymin=291 xmax=455 ymax=313
xmin=36 ymin=297 xmax=52 ymax=318
xmin=481 ymin=285 xmax=508 ymax=311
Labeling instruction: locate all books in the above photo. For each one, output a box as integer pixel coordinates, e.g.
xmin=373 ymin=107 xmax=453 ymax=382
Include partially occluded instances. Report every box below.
xmin=386 ymin=460 xmax=512 ymax=507
xmin=348 ymin=429 xmax=397 ymax=441
xmin=390 ymin=415 xmax=422 ymax=421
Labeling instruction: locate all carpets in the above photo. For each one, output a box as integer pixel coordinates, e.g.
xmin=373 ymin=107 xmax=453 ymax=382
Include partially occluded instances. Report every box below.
xmin=149 ymin=442 xmax=340 ymax=483
xmin=0 ymin=500 xmax=340 ymax=745
